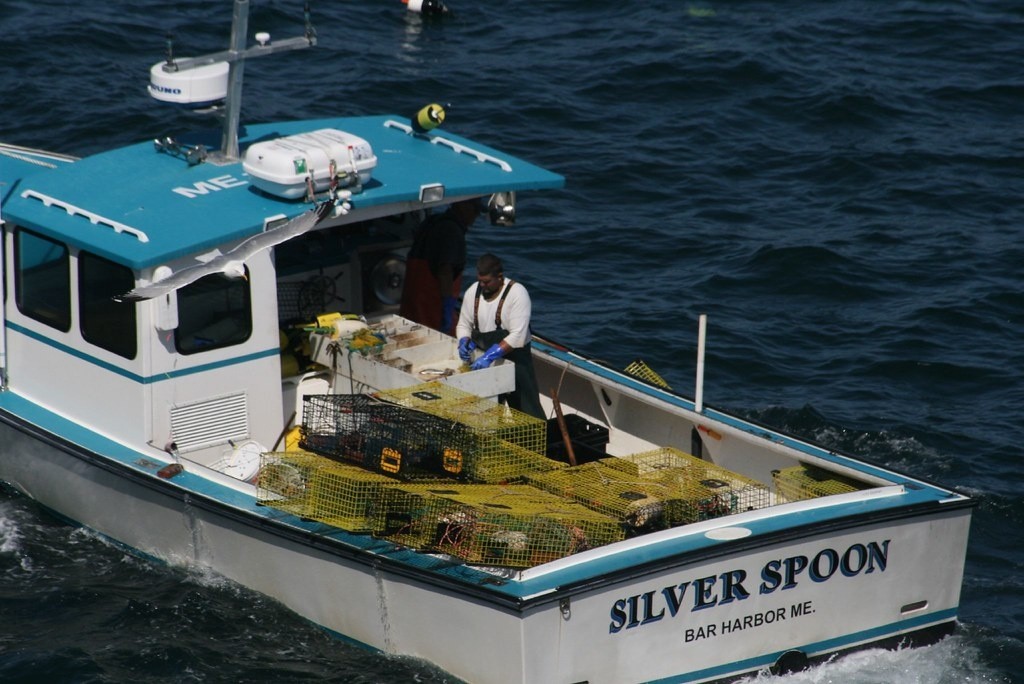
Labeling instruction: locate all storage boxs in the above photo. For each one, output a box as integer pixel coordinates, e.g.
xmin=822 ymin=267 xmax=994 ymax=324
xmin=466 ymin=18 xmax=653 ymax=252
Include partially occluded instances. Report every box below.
xmin=255 ymin=313 xmax=865 ymax=571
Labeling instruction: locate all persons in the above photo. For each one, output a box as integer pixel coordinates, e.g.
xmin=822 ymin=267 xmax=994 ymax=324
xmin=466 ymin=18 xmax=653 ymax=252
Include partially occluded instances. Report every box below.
xmin=399 ymin=195 xmax=489 ymax=337
xmin=456 ymin=255 xmax=549 ymax=421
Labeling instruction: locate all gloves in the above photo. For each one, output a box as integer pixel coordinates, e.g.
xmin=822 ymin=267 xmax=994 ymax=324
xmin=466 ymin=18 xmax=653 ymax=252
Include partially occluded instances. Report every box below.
xmin=458 ymin=336 xmax=475 ymax=362
xmin=470 ymin=344 xmax=504 ymax=371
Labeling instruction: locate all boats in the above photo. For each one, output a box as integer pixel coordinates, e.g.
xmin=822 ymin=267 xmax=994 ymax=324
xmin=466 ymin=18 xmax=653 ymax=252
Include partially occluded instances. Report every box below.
xmin=0 ymin=0 xmax=977 ymax=683
